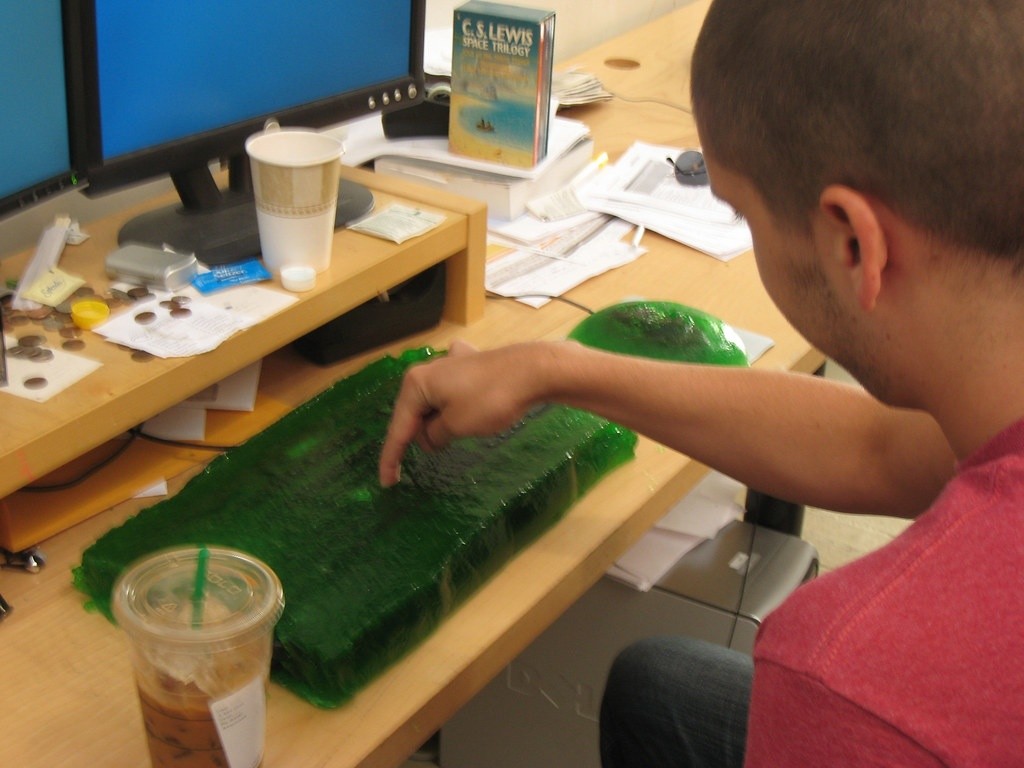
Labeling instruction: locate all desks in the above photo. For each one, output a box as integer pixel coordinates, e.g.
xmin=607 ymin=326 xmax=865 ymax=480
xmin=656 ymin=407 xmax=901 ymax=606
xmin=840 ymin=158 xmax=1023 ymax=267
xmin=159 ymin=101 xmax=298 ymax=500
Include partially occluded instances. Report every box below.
xmin=0 ymin=0 xmax=826 ymax=768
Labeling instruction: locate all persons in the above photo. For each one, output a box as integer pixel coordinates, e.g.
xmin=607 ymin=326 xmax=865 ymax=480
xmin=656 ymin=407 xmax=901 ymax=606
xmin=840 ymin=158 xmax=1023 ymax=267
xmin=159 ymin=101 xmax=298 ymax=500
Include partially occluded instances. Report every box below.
xmin=380 ymin=0 xmax=1024 ymax=768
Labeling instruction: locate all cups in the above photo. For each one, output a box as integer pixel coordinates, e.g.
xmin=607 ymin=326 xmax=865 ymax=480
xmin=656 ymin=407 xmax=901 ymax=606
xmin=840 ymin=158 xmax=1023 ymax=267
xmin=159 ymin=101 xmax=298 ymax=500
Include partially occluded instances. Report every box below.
xmin=245 ymin=127 xmax=346 ymax=275
xmin=109 ymin=544 xmax=287 ymax=768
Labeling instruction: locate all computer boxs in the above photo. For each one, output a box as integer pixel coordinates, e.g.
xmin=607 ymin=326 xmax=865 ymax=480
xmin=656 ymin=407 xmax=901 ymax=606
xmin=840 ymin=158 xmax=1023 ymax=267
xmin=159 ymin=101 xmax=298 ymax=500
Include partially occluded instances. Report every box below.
xmin=437 ymin=521 xmax=820 ymax=768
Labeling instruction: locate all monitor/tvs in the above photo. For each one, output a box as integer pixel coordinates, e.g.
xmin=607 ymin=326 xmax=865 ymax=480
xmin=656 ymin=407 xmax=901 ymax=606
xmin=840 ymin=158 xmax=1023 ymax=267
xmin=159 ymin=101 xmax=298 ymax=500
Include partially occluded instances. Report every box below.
xmin=59 ymin=0 xmax=427 ymax=265
